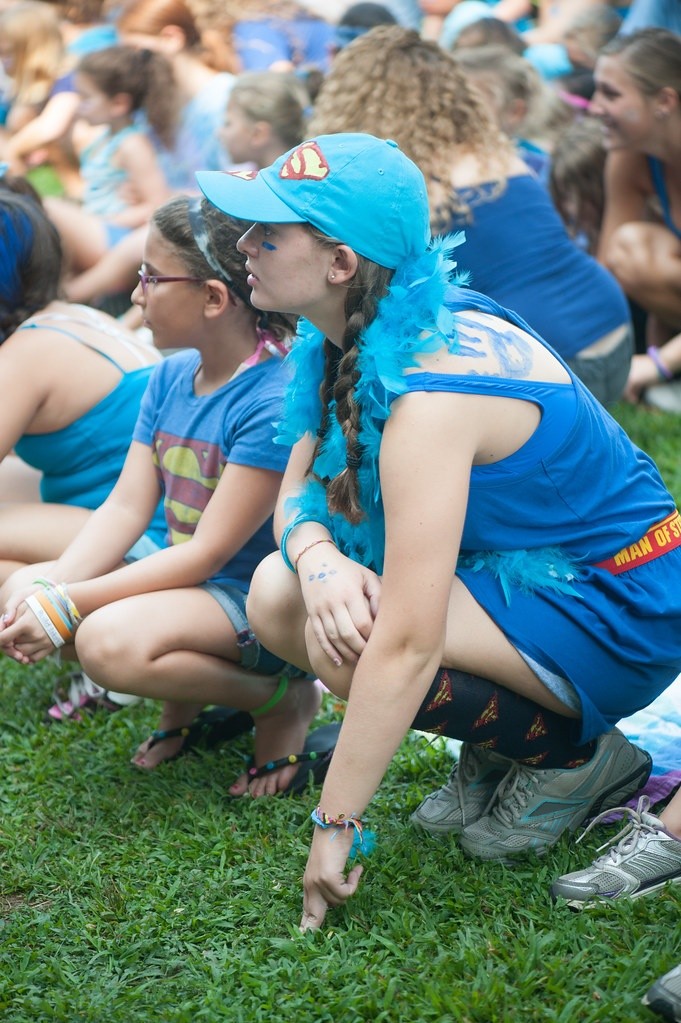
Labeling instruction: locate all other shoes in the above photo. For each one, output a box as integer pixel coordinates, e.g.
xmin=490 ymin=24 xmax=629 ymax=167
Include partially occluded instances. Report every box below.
xmin=645 ymin=379 xmax=681 ymax=416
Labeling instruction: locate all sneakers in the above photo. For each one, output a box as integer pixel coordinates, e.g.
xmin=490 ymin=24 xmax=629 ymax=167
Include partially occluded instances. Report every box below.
xmin=459 ymin=726 xmax=653 ymax=864
xmin=48 ymin=671 xmax=138 ymax=720
xmin=551 ymin=795 xmax=681 ymax=911
xmin=410 ymin=740 xmax=516 ymax=832
xmin=642 ymin=964 xmax=681 ymax=1023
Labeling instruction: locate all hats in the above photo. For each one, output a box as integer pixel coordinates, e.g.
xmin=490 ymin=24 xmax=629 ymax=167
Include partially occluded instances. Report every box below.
xmin=194 ymin=132 xmax=431 ymax=269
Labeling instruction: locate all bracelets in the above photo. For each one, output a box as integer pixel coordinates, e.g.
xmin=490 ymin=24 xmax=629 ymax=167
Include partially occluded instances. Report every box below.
xmin=294 ymin=540 xmax=337 ymax=574
xmin=648 ymin=345 xmax=672 ymax=382
xmin=310 ymin=808 xmax=374 ymax=863
xmin=280 ymin=513 xmax=337 ymax=574
xmin=25 ymin=583 xmax=81 ymax=647
xmin=33 ymin=579 xmax=49 ymax=587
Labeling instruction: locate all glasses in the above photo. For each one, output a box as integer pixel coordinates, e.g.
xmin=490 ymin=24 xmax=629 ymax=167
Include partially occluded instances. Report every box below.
xmin=139 ymin=270 xmax=238 ymax=308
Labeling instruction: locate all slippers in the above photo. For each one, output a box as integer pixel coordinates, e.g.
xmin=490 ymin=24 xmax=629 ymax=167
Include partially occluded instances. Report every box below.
xmin=246 ymin=724 xmax=341 ymax=799
xmin=147 ymin=704 xmax=256 ymax=762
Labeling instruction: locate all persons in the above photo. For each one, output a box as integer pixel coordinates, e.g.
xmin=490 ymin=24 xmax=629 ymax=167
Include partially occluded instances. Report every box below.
xmin=195 ymin=134 xmax=681 ymax=942
xmin=0 ymin=195 xmax=341 ymax=801
xmin=553 ymin=783 xmax=680 ymax=1023
xmin=1 ymin=1 xmax=680 ymax=582
